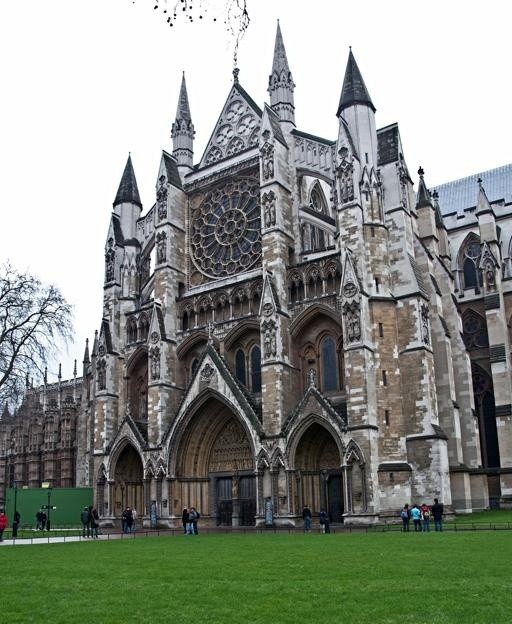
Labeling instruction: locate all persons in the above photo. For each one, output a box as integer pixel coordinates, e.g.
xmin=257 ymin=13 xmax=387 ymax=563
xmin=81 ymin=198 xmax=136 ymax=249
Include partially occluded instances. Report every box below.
xmin=301 ymin=502 xmax=311 ymax=530
xmin=401 ymin=504 xmax=411 ymax=531
xmin=431 ymin=498 xmax=443 ymax=531
xmin=1 ymin=505 xmax=8 ymax=542
xmin=87 ymin=505 xmax=100 ymax=538
xmin=410 ymin=504 xmax=422 ymax=531
xmin=182 ymin=508 xmax=190 ymax=533
xmin=318 ymin=506 xmax=331 ymax=531
xmin=124 ymin=506 xmax=133 ymax=531
xmin=42 ymin=511 xmax=48 ymax=529
xmin=81 ymin=506 xmax=89 ymax=537
xmin=419 ymin=503 xmax=430 ymax=532
xmin=36 ymin=509 xmax=43 ymax=531
xmin=131 ymin=507 xmax=138 ymax=530
xmin=187 ymin=507 xmax=199 ymax=534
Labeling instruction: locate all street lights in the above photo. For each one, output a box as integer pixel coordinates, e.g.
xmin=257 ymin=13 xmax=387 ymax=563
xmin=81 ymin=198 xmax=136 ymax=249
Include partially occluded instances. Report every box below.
xmin=13 ymin=480 xmax=19 ymax=536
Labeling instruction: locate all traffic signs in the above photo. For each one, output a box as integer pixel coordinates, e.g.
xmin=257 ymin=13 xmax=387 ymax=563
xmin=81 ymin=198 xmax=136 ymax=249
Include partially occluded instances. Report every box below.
xmin=46 ymin=480 xmax=54 ymax=532
